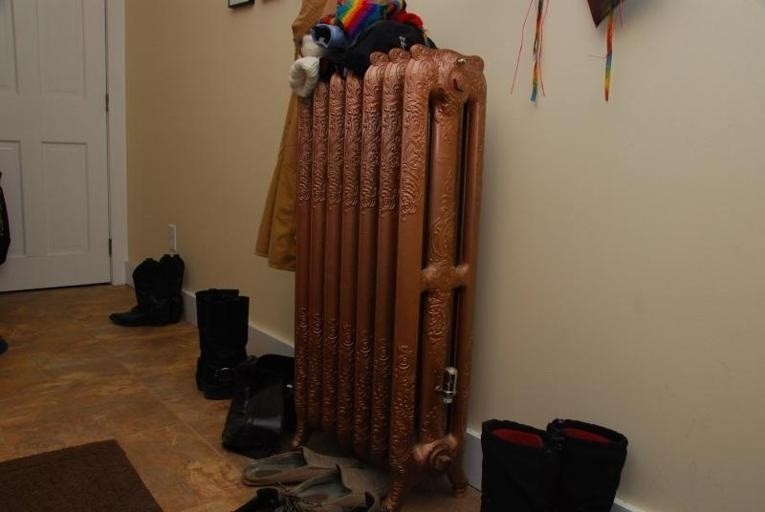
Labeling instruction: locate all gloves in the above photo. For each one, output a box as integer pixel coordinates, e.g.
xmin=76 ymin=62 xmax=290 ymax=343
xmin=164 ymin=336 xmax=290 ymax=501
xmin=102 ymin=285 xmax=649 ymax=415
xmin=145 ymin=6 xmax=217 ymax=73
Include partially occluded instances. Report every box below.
xmin=289 ymin=0 xmax=437 ymax=99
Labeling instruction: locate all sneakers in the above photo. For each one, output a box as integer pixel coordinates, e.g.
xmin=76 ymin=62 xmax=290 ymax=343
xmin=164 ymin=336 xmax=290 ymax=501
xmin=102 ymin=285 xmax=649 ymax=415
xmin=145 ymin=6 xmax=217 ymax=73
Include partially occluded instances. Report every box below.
xmin=242 ymin=445 xmax=387 ymax=510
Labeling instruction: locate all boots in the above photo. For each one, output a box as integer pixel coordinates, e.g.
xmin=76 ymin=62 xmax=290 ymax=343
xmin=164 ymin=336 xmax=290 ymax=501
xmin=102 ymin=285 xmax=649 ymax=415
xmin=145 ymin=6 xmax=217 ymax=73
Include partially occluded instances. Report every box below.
xmin=109 ymin=254 xmax=185 ymax=327
xmin=195 ymin=288 xmax=256 ymax=399
xmin=480 ymin=417 xmax=628 ymax=511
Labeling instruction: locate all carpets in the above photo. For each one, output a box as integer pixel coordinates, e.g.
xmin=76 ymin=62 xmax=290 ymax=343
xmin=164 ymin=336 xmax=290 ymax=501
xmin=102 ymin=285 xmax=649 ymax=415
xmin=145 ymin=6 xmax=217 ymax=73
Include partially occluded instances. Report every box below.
xmin=1 ymin=437 xmax=164 ymax=512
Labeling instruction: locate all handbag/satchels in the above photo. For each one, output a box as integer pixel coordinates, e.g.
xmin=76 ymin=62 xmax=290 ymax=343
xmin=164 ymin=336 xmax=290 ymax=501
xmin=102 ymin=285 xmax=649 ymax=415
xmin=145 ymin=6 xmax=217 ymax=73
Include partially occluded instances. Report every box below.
xmin=222 ymin=353 xmax=297 ymax=459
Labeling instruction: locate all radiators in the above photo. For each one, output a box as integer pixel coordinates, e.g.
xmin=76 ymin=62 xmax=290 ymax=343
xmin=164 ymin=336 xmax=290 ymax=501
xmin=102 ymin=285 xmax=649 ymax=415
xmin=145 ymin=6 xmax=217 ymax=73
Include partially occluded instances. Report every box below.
xmin=292 ymin=41 xmax=488 ymax=512
